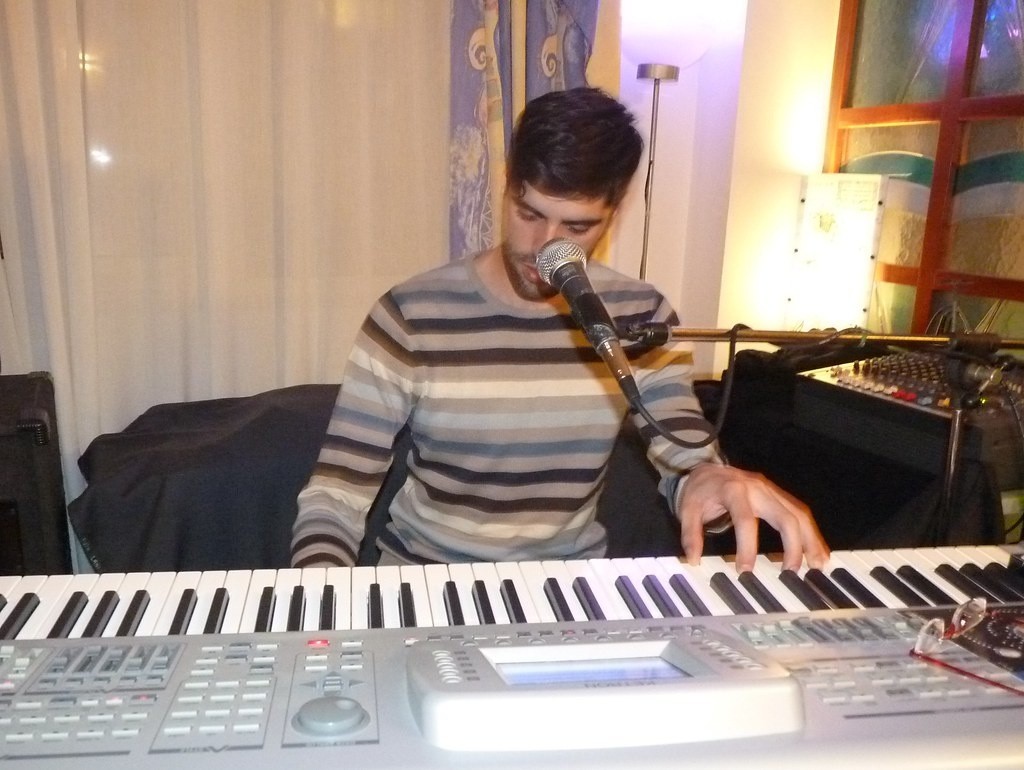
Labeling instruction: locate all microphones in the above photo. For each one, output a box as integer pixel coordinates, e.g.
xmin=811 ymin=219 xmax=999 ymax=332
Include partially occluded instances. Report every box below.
xmin=537 ymin=237 xmax=642 ymax=404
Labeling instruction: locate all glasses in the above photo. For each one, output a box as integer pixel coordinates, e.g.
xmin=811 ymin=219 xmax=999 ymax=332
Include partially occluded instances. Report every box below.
xmin=910 ymin=596 xmax=1024 ymax=696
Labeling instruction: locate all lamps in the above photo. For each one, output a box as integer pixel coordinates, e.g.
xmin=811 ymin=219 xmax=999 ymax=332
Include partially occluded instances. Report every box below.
xmin=636 ymin=63 xmax=680 ymax=281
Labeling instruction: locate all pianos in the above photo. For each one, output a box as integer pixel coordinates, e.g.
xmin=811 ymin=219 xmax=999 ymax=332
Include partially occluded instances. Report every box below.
xmin=1 ymin=541 xmax=1024 ymax=770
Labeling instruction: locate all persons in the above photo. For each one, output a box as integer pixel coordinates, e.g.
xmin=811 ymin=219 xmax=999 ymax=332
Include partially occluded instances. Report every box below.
xmin=288 ymin=87 xmax=830 ymax=574
